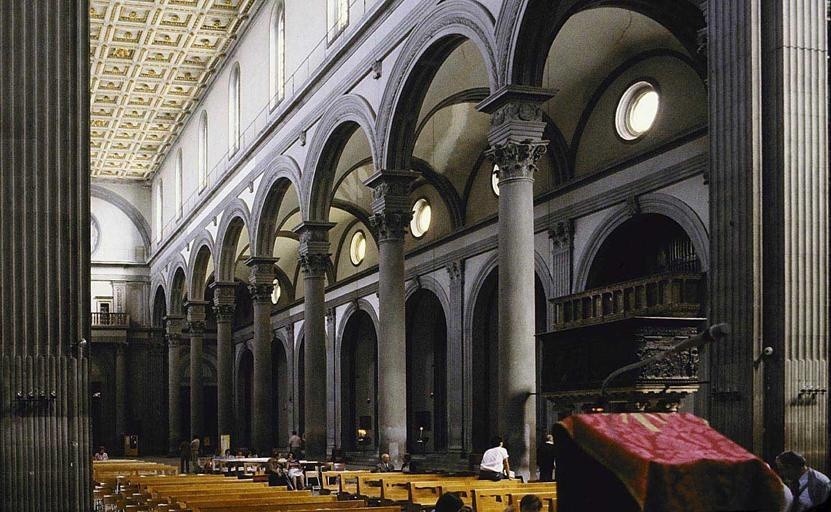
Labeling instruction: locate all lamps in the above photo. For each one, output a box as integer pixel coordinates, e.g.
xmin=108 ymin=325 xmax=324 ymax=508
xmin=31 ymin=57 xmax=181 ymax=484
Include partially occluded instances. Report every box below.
xmin=753 ymin=346 xmax=773 ymax=370
xmin=798 ymin=385 xmax=827 ymax=398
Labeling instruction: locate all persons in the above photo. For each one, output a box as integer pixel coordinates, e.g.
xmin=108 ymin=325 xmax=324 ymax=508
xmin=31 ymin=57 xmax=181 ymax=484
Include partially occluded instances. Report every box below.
xmin=178 ymin=430 xmax=306 ymax=492
xmin=401 ymin=454 xmax=416 ymax=473
xmin=95 ymin=445 xmax=109 ymax=461
xmin=536 ymin=434 xmax=555 ymax=481
xmin=775 ymin=450 xmax=831 ymax=512
xmin=476 ymin=436 xmax=513 ymax=481
xmin=504 ymin=495 xmax=543 ymax=512
xmin=431 ymin=492 xmax=464 ymax=512
xmin=376 ymin=453 xmax=394 ymax=472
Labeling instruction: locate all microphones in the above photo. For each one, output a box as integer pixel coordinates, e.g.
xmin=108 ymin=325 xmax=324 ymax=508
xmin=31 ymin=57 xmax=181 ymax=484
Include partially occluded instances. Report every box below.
xmin=580 ymin=323 xmax=732 ymax=414
